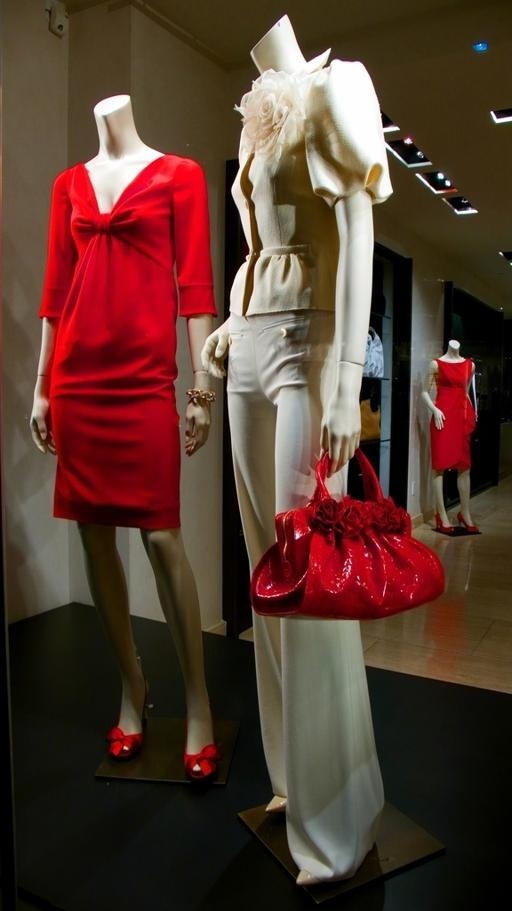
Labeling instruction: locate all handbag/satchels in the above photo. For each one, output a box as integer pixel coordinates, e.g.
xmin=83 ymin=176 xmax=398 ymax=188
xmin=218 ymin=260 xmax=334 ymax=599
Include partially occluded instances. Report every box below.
xmin=247 ymin=445 xmax=447 ymax=621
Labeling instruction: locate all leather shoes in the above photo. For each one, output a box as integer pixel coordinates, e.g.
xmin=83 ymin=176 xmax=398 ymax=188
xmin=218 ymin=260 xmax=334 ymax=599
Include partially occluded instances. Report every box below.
xmin=106 ymin=680 xmax=150 ymax=758
xmin=181 ymin=715 xmax=217 ymax=779
xmin=266 ymin=794 xmax=289 ymax=812
xmin=296 ymin=871 xmax=316 ymax=886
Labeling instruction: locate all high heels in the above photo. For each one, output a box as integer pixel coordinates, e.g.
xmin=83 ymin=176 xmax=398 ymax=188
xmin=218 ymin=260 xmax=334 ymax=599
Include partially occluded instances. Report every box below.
xmin=457 ymin=511 xmax=480 ymax=533
xmin=435 ymin=512 xmax=453 ymax=533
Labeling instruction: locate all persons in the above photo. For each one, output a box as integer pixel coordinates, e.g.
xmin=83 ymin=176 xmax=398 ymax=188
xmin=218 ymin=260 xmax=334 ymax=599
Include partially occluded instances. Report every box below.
xmin=420 ymin=339 xmax=482 ymax=535
xmin=197 ymin=11 xmax=401 ymax=887
xmin=29 ymin=92 xmax=220 ymax=782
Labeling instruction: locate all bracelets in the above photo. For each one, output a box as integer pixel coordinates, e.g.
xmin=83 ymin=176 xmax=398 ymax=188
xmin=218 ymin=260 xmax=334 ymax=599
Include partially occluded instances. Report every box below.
xmin=185 ymin=387 xmax=217 ymax=404
